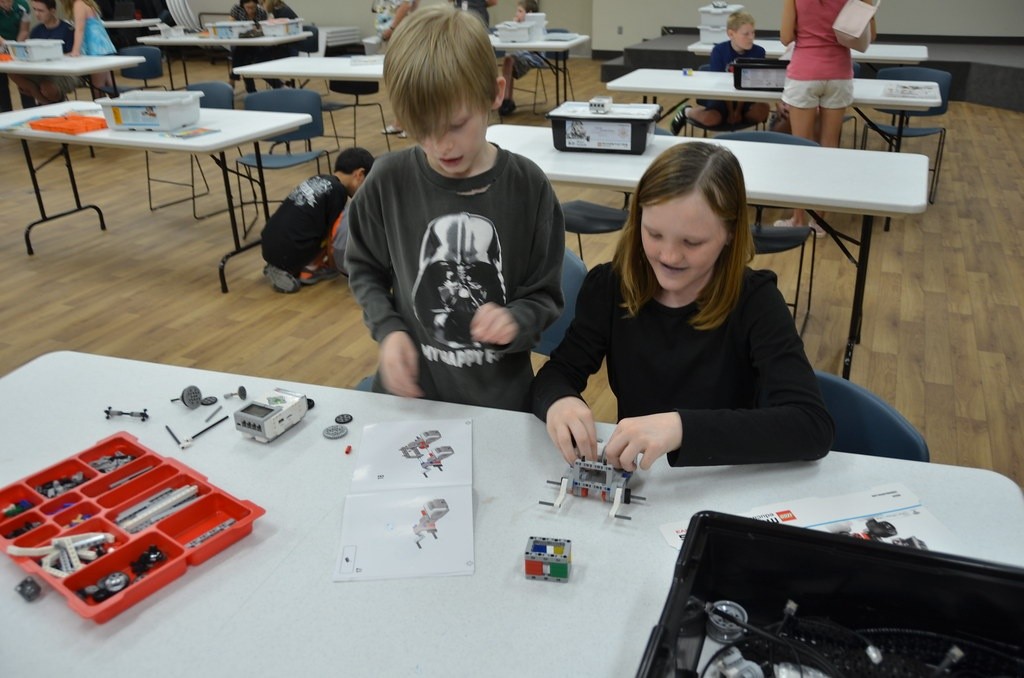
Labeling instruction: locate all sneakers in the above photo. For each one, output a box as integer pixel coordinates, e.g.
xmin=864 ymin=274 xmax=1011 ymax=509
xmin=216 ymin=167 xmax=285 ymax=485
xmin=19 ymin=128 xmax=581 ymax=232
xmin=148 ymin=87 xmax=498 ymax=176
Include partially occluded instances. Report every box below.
xmin=670 ymin=105 xmax=691 ymax=135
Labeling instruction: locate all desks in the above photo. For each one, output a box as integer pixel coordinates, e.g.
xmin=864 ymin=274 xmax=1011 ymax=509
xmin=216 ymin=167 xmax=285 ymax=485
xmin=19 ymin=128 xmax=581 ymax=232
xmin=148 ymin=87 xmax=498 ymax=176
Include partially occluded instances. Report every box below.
xmin=607 ymin=67 xmax=943 ymax=230
xmin=0 ymin=56 xmax=147 ymax=102
xmin=688 ymin=39 xmax=928 ymax=126
xmin=489 ymin=34 xmax=592 ymax=108
xmin=104 ymin=18 xmax=163 ymax=29
xmin=232 ymin=55 xmax=384 ymax=153
xmin=0 ymin=105 xmax=313 ymax=293
xmin=136 ymin=31 xmax=314 ymax=92
xmin=0 ymin=346 xmax=1024 ymax=678
xmin=485 ymin=124 xmax=930 ymax=381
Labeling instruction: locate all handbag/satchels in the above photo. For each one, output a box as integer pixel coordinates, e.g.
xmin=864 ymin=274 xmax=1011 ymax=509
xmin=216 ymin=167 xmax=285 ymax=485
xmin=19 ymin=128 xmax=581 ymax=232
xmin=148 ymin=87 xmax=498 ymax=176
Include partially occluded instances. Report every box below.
xmin=833 ymin=0 xmax=881 ymax=53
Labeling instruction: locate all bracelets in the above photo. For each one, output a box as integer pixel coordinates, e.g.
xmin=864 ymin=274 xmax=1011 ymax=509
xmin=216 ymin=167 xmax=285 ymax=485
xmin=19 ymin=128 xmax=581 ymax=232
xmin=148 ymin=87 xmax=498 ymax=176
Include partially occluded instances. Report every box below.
xmin=389 ymin=26 xmax=394 ymax=30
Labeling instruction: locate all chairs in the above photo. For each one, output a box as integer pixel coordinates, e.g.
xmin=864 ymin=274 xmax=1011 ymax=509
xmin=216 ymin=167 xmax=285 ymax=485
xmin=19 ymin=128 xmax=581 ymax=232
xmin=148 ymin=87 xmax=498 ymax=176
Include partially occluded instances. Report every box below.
xmin=489 ymin=26 xmax=576 ymax=114
xmin=841 ymin=60 xmax=951 ymax=205
xmin=97 ymin=26 xmax=391 ymax=242
xmin=530 ymin=127 xmax=928 ymax=466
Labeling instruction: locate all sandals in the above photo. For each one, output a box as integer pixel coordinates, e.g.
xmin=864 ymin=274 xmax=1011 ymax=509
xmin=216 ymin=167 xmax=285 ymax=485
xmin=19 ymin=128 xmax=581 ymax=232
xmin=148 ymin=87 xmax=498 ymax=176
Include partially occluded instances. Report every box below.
xmin=381 ymin=124 xmax=402 ymax=133
xmin=397 ymin=131 xmax=407 ymax=138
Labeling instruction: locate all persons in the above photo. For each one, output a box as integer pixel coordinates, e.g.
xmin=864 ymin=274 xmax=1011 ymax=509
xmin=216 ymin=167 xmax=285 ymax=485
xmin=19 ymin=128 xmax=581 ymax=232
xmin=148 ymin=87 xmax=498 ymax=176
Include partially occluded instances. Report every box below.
xmin=340 ymin=4 xmax=566 ymax=415
xmin=525 ymin=137 xmax=834 ymax=471
xmin=228 ymin=0 xmax=301 ymax=90
xmin=669 ymin=10 xmax=771 ymax=135
xmin=259 ymin=146 xmax=375 ymax=293
xmin=372 ymin=0 xmax=420 ymax=139
xmin=448 ymin=0 xmax=497 ymax=29
xmin=8 ymin=0 xmax=86 ymax=105
xmin=0 ymin=0 xmax=36 ymax=115
xmin=60 ymin=0 xmax=118 ymax=99
xmin=496 ymin=0 xmax=548 ymax=117
xmin=773 ymin=0 xmax=882 ymax=238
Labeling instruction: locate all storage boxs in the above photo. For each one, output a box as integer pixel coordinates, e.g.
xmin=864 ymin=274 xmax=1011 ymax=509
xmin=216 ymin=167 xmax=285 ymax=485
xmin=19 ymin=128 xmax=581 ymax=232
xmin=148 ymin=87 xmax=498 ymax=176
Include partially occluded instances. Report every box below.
xmin=697 ymin=4 xmax=744 ymax=44
xmin=495 ymin=20 xmax=549 ymax=42
xmin=157 ymin=21 xmax=186 ymax=37
xmin=260 ymin=17 xmax=304 ymax=38
xmin=635 ymin=510 xmax=1023 ymax=678
xmin=727 ymin=56 xmax=789 ymax=92
xmin=0 ymin=430 xmax=267 ymax=624
xmin=99 ymin=89 xmax=205 ymax=132
xmin=204 ymin=19 xmax=255 ymax=38
xmin=545 ymin=101 xmax=665 ymax=152
xmin=6 ymin=38 xmax=67 ymax=64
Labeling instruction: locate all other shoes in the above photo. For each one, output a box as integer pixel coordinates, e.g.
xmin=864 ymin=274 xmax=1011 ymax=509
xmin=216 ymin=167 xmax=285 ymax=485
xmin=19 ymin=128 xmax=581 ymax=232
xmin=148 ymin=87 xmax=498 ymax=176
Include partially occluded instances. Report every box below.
xmin=798 ymin=220 xmax=827 ymax=239
xmin=299 ymin=267 xmax=338 ymax=284
xmin=500 ymin=99 xmax=514 ymax=115
xmin=774 ymin=219 xmax=792 ymax=227
xmin=264 ymin=264 xmax=299 ymax=292
